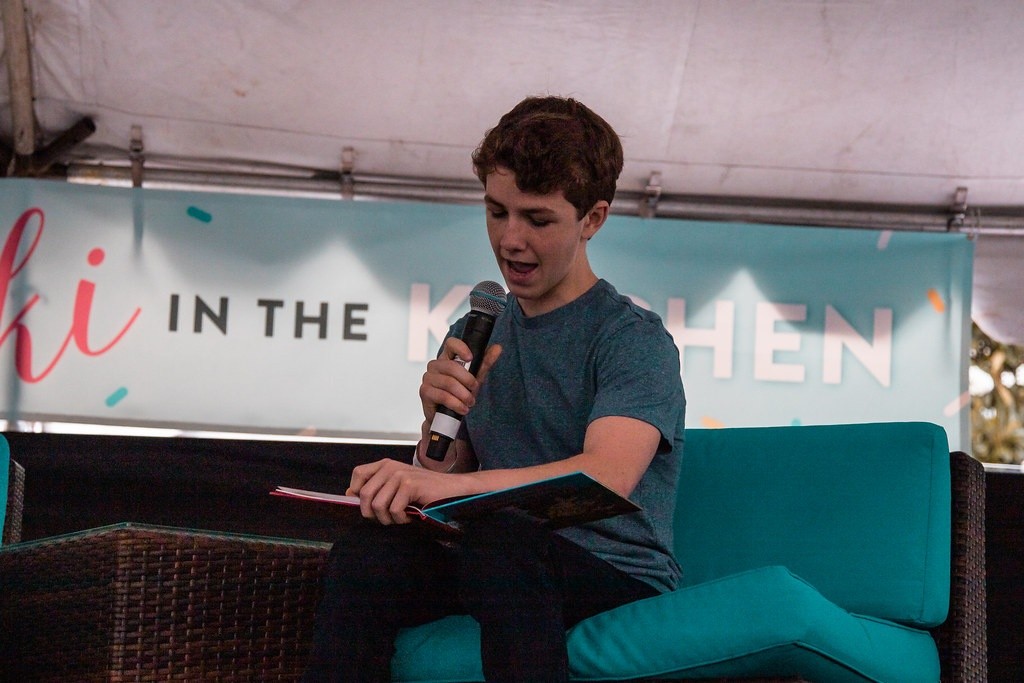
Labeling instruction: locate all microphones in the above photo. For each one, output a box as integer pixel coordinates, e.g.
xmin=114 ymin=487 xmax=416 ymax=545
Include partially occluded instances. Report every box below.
xmin=424 ymin=281 xmax=507 ymax=461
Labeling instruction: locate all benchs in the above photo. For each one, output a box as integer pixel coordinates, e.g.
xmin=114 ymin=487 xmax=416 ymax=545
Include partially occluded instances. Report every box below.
xmin=388 ymin=421 xmax=988 ymax=683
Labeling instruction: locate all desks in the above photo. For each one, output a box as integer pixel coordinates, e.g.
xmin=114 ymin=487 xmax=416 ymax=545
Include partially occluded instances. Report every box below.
xmin=1 ymin=523 xmax=335 ymax=682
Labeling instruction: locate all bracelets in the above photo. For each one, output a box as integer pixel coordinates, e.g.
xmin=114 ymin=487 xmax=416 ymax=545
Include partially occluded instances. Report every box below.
xmin=413 ymin=437 xmax=460 ymax=473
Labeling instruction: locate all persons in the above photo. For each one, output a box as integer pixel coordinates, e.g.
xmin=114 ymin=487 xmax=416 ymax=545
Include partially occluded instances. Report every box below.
xmin=306 ymin=96 xmax=685 ymax=683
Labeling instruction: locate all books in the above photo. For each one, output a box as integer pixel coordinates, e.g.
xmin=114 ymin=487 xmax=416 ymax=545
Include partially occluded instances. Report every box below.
xmin=269 ymin=472 xmax=643 ymax=539
